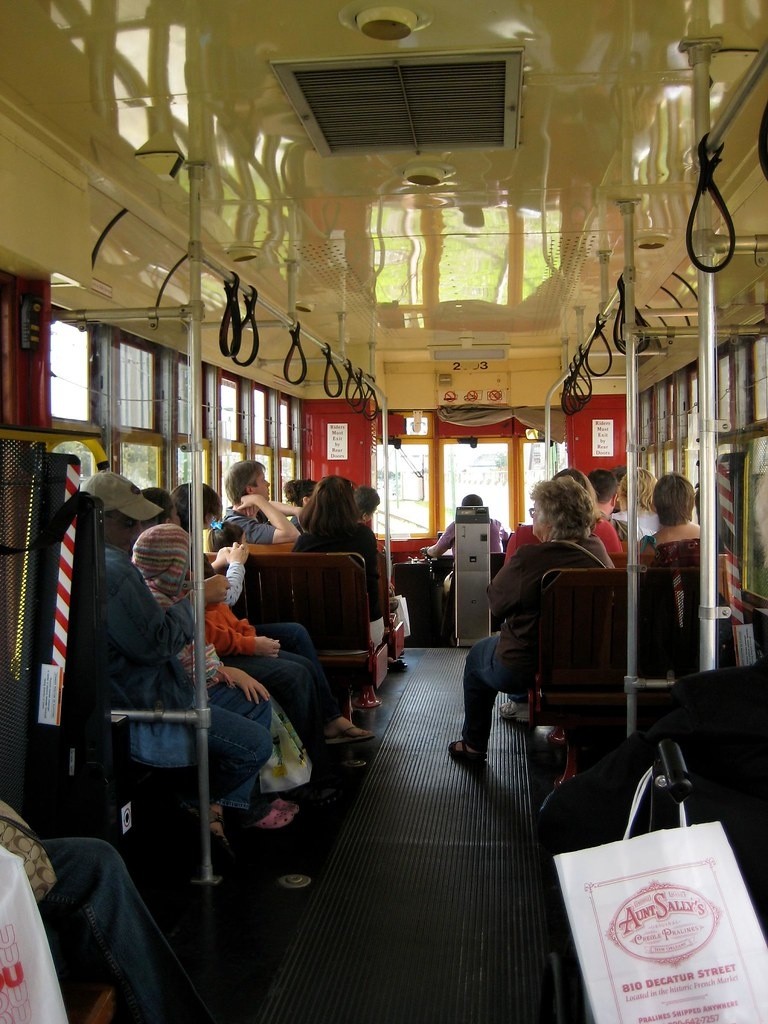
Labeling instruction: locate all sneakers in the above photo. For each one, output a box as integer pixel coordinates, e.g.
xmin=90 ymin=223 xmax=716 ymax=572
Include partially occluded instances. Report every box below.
xmin=500 ymin=700 xmax=528 ymax=722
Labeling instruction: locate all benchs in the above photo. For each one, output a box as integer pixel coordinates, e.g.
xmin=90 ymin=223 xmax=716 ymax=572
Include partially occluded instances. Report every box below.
xmin=207 ymin=551 xmax=389 ymax=722
xmin=527 ymin=567 xmax=735 ymax=790
xmin=246 ymin=543 xmax=405 ymax=660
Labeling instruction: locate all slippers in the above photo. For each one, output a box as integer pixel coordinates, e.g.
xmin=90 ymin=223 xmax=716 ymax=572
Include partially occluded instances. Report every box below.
xmin=325 ymin=726 xmax=375 ymax=744
xmin=449 ymin=740 xmax=487 ymax=759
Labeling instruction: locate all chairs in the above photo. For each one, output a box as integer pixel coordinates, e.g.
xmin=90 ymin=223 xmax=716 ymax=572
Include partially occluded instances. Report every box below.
xmin=450 ymin=553 xmax=506 ymax=647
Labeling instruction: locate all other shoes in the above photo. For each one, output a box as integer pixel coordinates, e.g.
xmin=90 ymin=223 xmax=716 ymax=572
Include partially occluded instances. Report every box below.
xmin=243 ymin=808 xmax=294 ymax=828
xmin=270 ymin=798 xmax=300 ymax=814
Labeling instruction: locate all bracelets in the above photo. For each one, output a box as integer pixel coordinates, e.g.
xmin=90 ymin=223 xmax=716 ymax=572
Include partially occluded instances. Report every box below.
xmin=423 ymin=547 xmax=434 ymax=559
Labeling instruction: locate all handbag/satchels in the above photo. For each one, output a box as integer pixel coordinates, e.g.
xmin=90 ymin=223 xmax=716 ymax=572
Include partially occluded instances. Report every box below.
xmin=0 ymin=799 xmax=58 ymax=903
xmin=0 ymin=844 xmax=69 ymax=1024
xmin=554 ymin=766 xmax=768 ymax=1024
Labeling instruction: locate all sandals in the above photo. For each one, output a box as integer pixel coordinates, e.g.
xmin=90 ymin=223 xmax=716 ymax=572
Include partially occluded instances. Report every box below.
xmin=181 ymin=802 xmax=235 ymax=864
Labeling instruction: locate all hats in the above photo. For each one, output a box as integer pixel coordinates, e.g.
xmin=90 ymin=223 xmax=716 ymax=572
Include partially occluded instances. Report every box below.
xmin=90 ymin=470 xmax=164 ymax=520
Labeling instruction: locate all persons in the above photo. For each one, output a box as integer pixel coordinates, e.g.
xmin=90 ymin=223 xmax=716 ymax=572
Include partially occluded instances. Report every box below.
xmin=419 ymin=463 xmax=732 ymax=771
xmin=68 ymin=458 xmax=410 ymax=851
xmin=534 ymin=653 xmax=767 ymax=859
xmin=28 ymin=836 xmax=216 ymax=1024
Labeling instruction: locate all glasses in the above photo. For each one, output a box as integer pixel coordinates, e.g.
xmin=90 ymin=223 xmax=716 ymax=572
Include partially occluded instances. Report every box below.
xmin=529 ymin=508 xmax=536 ymax=518
xmin=104 ymin=513 xmax=138 ymax=529
